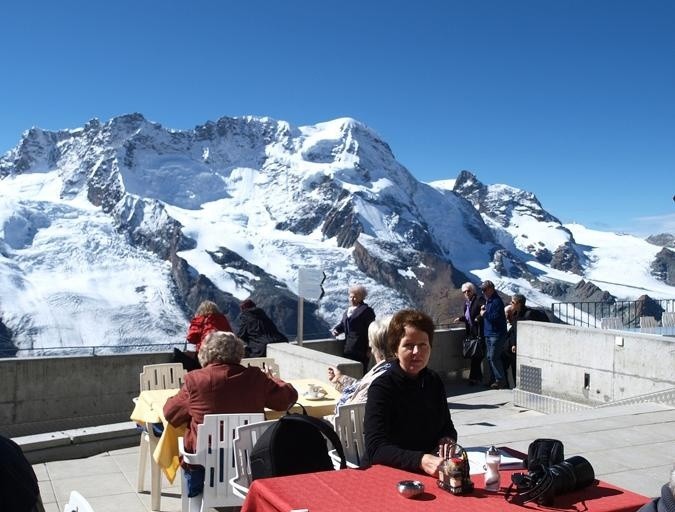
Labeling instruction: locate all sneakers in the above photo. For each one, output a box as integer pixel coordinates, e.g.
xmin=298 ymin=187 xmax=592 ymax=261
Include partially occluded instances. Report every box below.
xmin=469 ymin=377 xmax=507 ymax=391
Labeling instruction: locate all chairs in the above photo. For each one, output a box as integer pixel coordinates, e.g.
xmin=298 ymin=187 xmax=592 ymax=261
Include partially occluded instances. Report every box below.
xmin=138 ymin=363 xmax=183 ymax=493
xmin=230 ymin=417 xmax=334 ymax=511
xmin=130 ymin=377 xmax=350 ymax=512
xmin=328 ymin=402 xmax=367 ymax=468
xmin=239 ymin=357 xmax=276 ymax=378
xmin=178 ymin=413 xmax=265 ymax=512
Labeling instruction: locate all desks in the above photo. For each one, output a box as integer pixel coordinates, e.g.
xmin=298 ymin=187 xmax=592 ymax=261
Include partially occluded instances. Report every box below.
xmin=242 ymin=447 xmax=652 ymax=512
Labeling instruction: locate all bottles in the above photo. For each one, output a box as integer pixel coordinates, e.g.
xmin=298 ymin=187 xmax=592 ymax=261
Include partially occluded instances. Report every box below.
xmin=484 ymin=446 xmax=503 ymax=491
xmin=438 ymin=457 xmax=464 ymax=495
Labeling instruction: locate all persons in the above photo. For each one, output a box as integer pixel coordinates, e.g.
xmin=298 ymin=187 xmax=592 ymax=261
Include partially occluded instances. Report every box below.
xmin=453 ymin=281 xmax=549 ymax=389
xmin=186 ymin=302 xmax=233 ymax=370
xmin=163 ymin=330 xmax=297 ymax=498
xmin=234 ymin=299 xmax=289 ymax=359
xmin=331 ymin=285 xmax=377 ymax=377
xmin=364 ymin=308 xmax=457 ymax=479
xmin=327 ymin=318 xmax=397 ymax=460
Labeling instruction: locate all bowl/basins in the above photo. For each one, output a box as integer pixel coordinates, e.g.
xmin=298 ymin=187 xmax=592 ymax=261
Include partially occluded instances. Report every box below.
xmin=395 ymin=480 xmax=425 ymax=499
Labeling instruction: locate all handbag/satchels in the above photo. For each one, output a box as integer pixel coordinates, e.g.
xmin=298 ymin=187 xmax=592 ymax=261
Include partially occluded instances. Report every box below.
xmin=170 ymin=339 xmax=202 ymax=372
xmin=462 ymin=336 xmax=484 ymax=361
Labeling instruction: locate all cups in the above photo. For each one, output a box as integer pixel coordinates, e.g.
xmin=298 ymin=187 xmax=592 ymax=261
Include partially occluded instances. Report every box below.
xmin=308 ymin=384 xmax=322 ymax=397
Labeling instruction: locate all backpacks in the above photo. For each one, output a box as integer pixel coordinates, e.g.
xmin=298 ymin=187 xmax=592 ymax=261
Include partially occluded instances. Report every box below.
xmin=249 ymin=401 xmax=347 ymax=480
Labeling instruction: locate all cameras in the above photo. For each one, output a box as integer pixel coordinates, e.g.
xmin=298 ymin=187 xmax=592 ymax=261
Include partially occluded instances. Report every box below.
xmin=509 ymin=439 xmax=596 ymax=506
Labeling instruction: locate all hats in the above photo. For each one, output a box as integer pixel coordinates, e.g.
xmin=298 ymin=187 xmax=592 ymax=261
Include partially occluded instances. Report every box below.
xmin=478 ymin=281 xmax=495 ymax=290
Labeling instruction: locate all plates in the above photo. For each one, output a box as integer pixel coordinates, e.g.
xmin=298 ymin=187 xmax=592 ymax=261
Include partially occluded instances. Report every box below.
xmin=302 ymin=391 xmax=328 ymax=400
